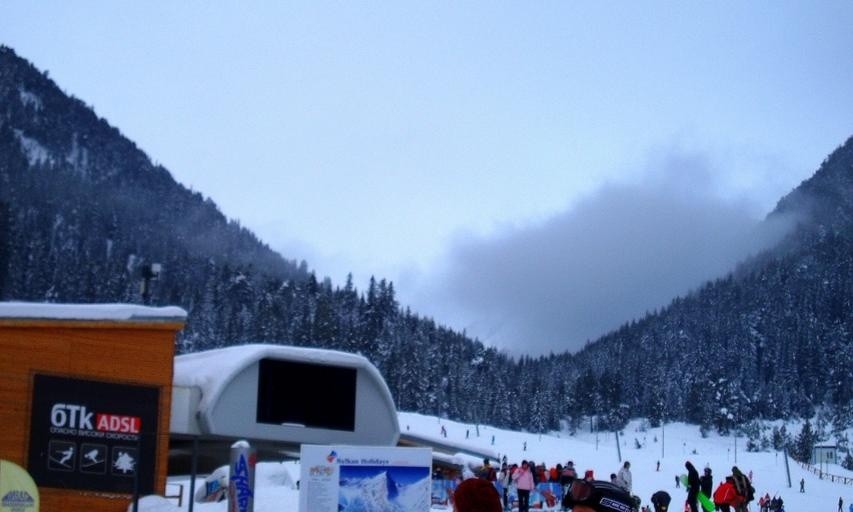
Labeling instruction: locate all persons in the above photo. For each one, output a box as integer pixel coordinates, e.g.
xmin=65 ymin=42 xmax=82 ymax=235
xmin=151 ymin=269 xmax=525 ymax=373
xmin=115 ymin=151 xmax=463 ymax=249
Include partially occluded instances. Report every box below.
xmin=849 ymin=504 xmax=853 ymax=512
xmin=838 ymin=497 xmax=842 ymax=512
xmin=800 ymin=477 xmax=805 ymax=492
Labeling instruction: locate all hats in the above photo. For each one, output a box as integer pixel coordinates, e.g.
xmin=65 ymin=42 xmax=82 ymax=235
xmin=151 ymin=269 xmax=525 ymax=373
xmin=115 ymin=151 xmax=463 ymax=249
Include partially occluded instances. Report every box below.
xmin=704 ymin=467 xmax=712 ymax=474
xmin=566 ymin=461 xmax=574 ymax=465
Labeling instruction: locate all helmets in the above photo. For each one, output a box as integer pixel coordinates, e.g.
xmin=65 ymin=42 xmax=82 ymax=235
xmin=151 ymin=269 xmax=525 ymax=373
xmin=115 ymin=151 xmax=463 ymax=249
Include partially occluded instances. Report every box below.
xmin=652 ymin=491 xmax=671 ymax=511
